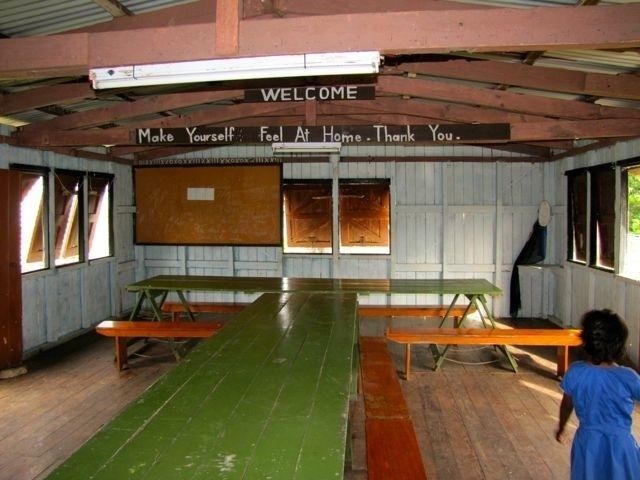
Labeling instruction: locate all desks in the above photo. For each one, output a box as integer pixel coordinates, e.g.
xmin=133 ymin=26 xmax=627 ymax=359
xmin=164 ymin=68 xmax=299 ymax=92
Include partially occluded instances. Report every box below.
xmin=44 ymin=289 xmax=359 ymax=480
xmin=123 ymin=271 xmax=519 ymax=376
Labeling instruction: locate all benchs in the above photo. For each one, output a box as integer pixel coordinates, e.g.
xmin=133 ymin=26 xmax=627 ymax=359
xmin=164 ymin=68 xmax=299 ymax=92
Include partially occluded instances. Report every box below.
xmin=355 ymin=300 xmax=475 ymax=333
xmin=160 ymin=300 xmax=248 ymax=341
xmin=94 ymin=319 xmax=224 ymax=376
xmin=378 ymin=323 xmax=584 ymax=383
xmin=356 ymin=335 xmax=427 ymax=480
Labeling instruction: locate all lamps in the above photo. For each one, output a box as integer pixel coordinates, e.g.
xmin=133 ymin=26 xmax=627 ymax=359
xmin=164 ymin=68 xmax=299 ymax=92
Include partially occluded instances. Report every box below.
xmin=271 ymin=140 xmax=343 ymax=156
xmin=90 ymin=52 xmax=380 ymax=93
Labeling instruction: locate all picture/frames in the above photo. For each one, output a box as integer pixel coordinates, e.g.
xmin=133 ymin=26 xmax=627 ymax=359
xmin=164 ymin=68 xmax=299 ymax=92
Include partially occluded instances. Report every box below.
xmin=131 ymin=161 xmax=284 ymax=248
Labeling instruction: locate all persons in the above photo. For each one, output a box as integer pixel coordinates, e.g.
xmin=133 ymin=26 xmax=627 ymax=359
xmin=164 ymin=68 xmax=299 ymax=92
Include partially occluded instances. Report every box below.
xmin=553 ymin=307 xmax=640 ymax=480
